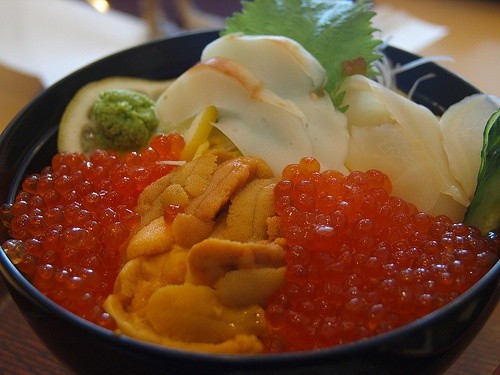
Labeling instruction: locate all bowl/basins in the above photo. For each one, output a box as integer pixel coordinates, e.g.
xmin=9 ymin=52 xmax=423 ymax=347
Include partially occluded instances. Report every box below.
xmin=2 ymin=21 xmax=499 ymax=374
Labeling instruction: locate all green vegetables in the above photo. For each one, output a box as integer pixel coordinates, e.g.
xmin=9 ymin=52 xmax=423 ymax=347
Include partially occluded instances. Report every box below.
xmin=218 ymin=0 xmax=385 ymax=112
